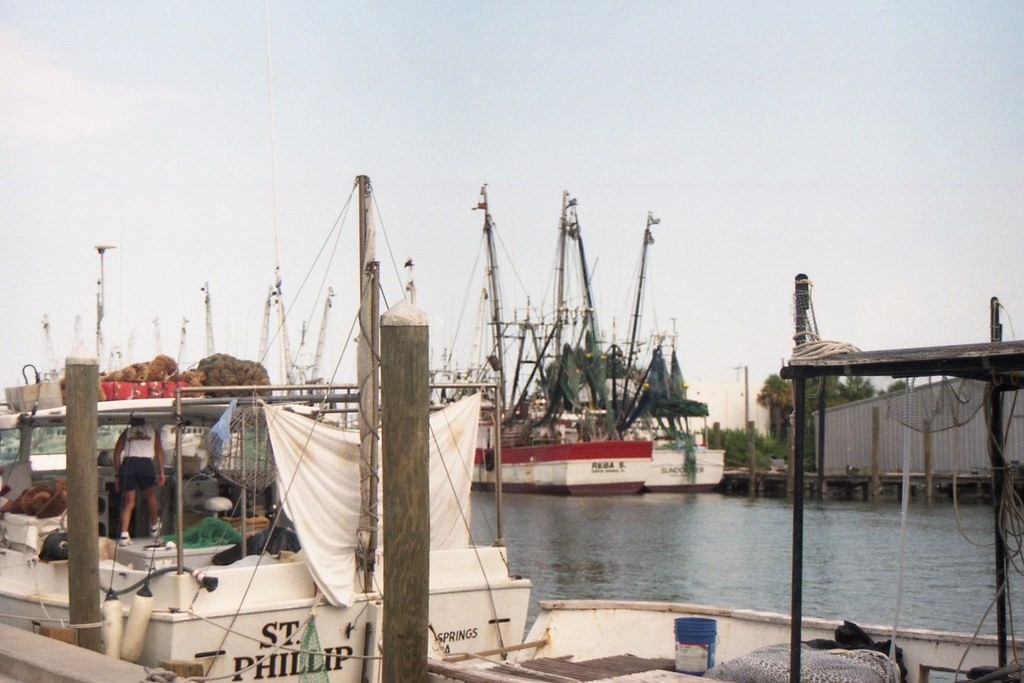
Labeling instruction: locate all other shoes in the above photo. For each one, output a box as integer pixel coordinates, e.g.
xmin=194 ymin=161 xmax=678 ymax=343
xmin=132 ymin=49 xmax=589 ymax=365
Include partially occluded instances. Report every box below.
xmin=119 ymin=536 xmax=135 ymax=546
xmin=150 ymin=517 xmax=162 ymax=538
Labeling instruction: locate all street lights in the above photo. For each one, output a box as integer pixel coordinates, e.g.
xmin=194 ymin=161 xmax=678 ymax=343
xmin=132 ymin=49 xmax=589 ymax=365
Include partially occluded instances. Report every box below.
xmin=97 ymin=245 xmax=120 ymax=368
xmin=734 ymin=366 xmax=749 ymax=451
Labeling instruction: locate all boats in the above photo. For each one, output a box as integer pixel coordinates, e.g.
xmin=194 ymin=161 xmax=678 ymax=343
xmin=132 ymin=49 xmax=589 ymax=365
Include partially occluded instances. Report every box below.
xmin=1 ymin=172 xmax=532 ymax=683
xmin=427 ymin=274 xmax=1024 ymax=683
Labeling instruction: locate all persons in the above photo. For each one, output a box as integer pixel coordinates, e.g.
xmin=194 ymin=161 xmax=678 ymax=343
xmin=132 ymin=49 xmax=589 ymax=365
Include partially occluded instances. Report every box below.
xmin=114 ymin=417 xmax=166 ymax=546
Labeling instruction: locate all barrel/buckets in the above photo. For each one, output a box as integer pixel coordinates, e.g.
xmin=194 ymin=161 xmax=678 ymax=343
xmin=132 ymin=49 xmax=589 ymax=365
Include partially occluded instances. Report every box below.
xmin=674 ymin=617 xmax=717 ymax=678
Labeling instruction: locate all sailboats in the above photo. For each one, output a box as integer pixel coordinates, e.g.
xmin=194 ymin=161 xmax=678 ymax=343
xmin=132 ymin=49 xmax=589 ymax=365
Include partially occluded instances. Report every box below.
xmin=473 ymin=185 xmax=722 ymax=496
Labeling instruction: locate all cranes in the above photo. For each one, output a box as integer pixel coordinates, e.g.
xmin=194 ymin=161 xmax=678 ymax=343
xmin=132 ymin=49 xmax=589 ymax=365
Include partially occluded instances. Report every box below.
xmin=200 ymin=281 xmax=214 ymax=358
xmin=260 ymin=266 xmax=336 ymax=405
xmin=257 ymin=7 xmax=294 ymax=391
xmin=149 ymin=314 xmax=190 ymax=371
